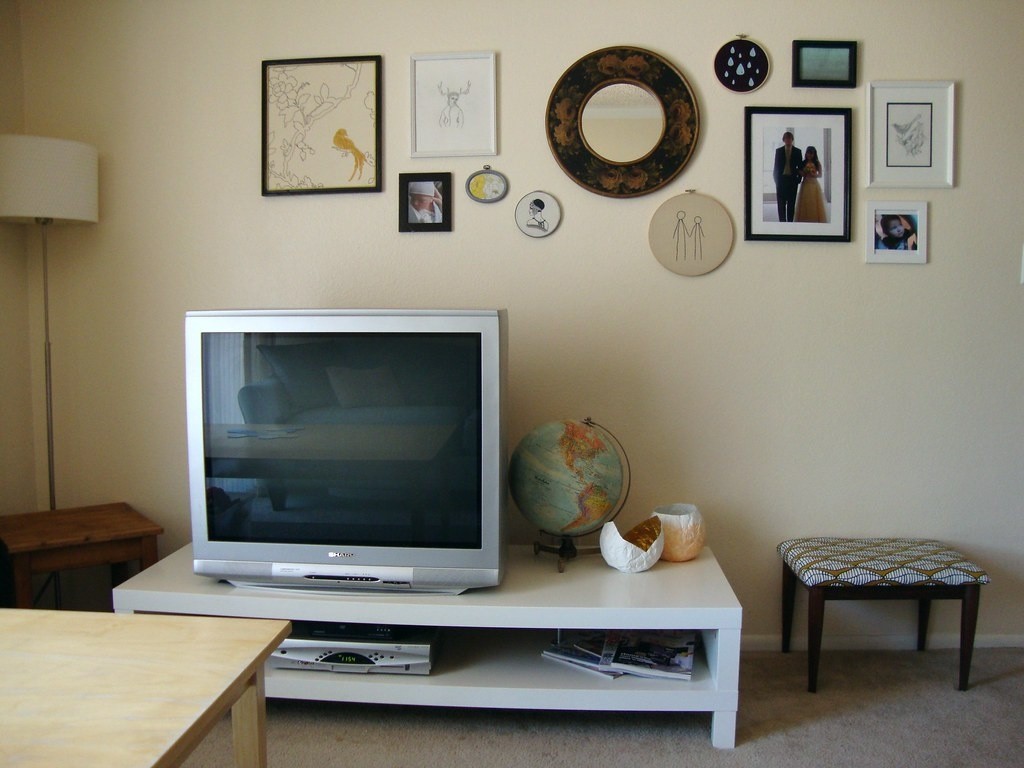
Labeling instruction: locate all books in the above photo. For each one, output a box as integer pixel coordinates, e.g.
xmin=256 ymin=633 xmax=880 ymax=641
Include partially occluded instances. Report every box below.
xmin=541 ymin=629 xmax=696 ymax=680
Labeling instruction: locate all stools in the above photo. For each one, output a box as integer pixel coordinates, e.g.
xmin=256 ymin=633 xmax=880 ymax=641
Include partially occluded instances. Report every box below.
xmin=777 ymin=538 xmax=990 ymax=694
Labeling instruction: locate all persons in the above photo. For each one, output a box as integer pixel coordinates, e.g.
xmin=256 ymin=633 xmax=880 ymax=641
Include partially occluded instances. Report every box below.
xmin=773 ymin=132 xmax=828 ymax=223
xmin=409 ymin=182 xmax=442 ymax=223
xmin=880 ymin=215 xmax=912 ymax=249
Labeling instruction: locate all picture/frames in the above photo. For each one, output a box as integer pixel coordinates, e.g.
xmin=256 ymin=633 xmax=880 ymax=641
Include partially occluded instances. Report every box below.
xmin=399 ymin=172 xmax=451 ymax=232
xmin=791 ymin=39 xmax=857 ymax=88
xmin=866 ymin=80 xmax=956 ymax=190
xmin=863 ymin=199 xmax=928 ymax=263
xmin=261 ymin=55 xmax=382 ymax=195
xmin=743 ymin=106 xmax=852 ymax=242
xmin=407 ymin=51 xmax=497 ymax=159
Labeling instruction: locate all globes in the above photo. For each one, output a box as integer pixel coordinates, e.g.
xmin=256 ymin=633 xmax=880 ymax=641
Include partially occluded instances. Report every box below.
xmin=508 ymin=416 xmax=632 ymax=573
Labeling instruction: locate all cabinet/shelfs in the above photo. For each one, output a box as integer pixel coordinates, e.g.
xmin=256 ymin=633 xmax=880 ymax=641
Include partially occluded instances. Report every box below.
xmin=111 ymin=539 xmax=741 ymax=749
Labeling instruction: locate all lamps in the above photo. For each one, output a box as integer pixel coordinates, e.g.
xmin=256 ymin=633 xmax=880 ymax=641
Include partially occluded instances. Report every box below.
xmin=0 ymin=131 xmax=100 ymax=612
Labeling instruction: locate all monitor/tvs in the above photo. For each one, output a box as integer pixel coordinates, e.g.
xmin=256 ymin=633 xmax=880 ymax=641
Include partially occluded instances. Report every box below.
xmin=186 ymin=308 xmax=511 ymax=595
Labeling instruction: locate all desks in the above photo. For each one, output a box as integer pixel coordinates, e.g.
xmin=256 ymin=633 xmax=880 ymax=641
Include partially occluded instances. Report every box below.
xmin=0 ymin=502 xmax=164 ymax=610
xmin=0 ymin=604 xmax=292 ymax=768
